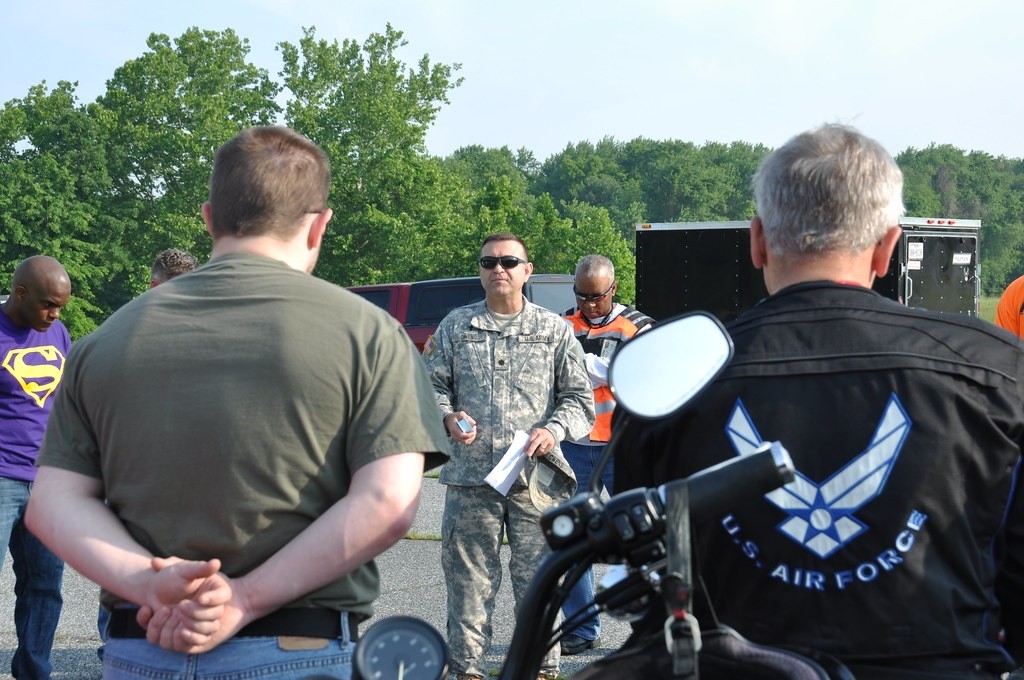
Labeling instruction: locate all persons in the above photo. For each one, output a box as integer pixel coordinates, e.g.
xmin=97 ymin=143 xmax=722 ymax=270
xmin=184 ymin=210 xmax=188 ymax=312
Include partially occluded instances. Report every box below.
xmin=610 ymin=121 xmax=1024 ymax=680
xmin=420 ymin=233 xmax=657 ymax=680
xmin=0 ymin=125 xmax=451 ymax=680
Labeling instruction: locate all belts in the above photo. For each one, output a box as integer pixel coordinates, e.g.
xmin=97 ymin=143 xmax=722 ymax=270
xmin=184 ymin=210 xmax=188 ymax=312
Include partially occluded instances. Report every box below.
xmin=108 ymin=606 xmax=362 ymax=643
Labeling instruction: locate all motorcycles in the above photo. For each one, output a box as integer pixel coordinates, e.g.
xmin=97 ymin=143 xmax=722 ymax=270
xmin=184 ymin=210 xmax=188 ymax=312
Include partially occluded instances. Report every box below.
xmin=351 ymin=309 xmax=835 ymax=680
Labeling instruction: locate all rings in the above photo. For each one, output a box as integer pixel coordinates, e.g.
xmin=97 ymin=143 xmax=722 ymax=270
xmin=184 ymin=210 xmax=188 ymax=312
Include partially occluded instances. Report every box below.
xmin=540 ymin=445 xmax=545 ymax=449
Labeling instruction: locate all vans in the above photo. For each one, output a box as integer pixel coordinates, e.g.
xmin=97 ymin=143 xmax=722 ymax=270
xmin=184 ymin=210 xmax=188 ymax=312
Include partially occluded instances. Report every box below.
xmin=343 ymin=273 xmax=575 ymax=356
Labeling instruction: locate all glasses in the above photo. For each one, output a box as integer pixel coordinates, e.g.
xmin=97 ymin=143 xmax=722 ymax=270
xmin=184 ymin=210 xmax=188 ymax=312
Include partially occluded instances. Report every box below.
xmin=573 ymin=281 xmax=614 ymax=302
xmin=478 ymin=256 xmax=526 ymax=270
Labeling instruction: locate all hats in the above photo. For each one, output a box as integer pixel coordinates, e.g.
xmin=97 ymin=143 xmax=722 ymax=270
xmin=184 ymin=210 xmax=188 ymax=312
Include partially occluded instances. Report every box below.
xmin=525 ymin=445 xmax=578 ymax=514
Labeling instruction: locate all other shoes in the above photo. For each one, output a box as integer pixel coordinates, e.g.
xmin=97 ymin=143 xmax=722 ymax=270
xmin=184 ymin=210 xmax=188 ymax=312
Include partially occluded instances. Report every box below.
xmin=560 ymin=634 xmax=601 ymax=654
